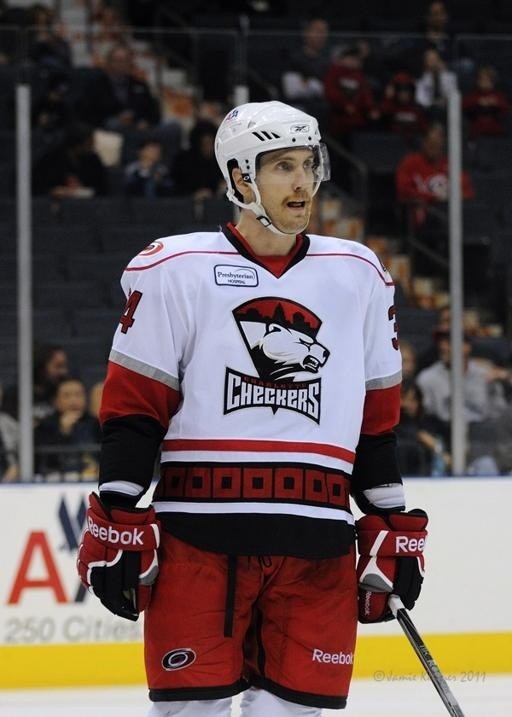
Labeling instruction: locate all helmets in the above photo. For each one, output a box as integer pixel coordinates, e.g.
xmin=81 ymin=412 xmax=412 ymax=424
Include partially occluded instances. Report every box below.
xmin=214 ymin=99 xmax=331 ymax=200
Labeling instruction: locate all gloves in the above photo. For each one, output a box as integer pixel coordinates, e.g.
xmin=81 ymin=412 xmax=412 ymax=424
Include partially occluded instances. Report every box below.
xmin=353 ymin=508 xmax=429 ymax=625
xmin=75 ymin=489 xmax=163 ymax=622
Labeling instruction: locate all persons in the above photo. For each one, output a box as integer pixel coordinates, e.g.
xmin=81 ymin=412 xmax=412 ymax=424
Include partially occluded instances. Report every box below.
xmin=1 ymin=0 xmax=512 ymax=483
xmin=76 ymin=100 xmax=428 ymax=717
